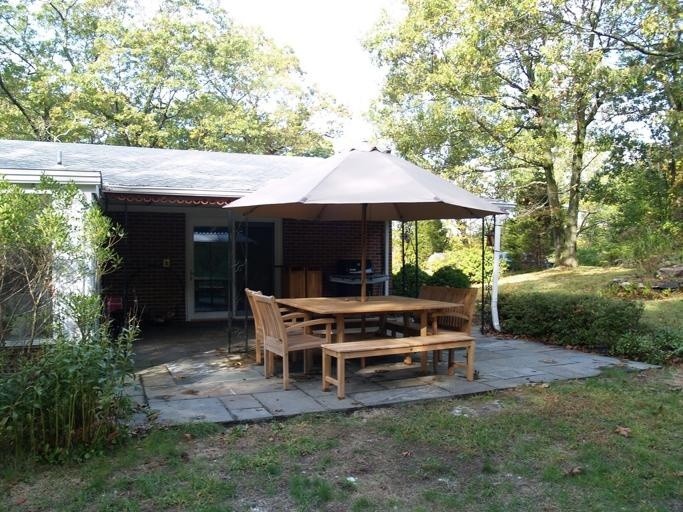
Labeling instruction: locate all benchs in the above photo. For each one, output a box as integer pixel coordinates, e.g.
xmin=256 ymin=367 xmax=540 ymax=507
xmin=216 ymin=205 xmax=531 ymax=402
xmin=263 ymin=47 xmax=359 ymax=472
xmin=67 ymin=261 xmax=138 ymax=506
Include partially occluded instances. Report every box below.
xmin=317 ymin=333 xmax=476 ymax=400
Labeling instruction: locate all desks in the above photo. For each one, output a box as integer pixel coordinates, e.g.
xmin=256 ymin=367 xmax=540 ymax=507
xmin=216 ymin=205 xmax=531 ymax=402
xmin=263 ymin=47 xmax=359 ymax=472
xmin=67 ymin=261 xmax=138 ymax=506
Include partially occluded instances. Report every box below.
xmin=272 ymin=295 xmax=464 ymax=384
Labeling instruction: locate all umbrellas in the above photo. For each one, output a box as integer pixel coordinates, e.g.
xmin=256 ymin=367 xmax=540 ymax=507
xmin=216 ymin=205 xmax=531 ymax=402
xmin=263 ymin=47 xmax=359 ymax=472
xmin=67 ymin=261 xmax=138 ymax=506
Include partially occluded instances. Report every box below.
xmin=220 ymin=137 xmax=511 ymax=371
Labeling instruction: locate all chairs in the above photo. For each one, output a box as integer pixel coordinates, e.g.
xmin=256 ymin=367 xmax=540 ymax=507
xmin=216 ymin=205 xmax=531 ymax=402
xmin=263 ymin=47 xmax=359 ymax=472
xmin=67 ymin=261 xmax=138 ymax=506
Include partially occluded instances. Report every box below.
xmin=242 ymin=287 xmax=336 ymax=391
xmin=383 ymin=285 xmax=478 ymax=360
